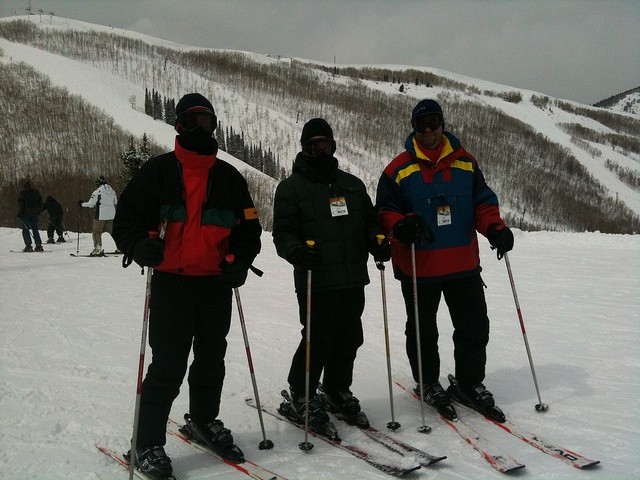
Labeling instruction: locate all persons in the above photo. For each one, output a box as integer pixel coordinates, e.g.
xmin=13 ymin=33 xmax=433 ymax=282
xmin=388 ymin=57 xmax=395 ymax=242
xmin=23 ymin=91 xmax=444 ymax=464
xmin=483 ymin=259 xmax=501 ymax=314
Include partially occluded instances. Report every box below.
xmin=377 ymin=99 xmax=513 ymax=407
xmin=17 ymin=178 xmax=44 ymax=252
xmin=113 ymin=94 xmax=261 ymax=477
xmin=272 ymin=118 xmax=391 ymax=440
xmin=79 ymin=175 xmax=123 ymax=257
xmin=42 ymin=195 xmax=66 ymax=244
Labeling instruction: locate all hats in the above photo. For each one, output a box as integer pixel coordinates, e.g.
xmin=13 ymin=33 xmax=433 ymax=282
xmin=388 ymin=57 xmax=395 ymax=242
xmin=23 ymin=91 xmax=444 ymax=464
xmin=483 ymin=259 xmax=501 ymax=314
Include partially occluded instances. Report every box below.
xmin=413 ymin=99 xmax=444 ymax=118
xmin=177 ymin=93 xmax=216 ymax=119
xmin=96 ymin=175 xmax=106 ymax=184
xmin=302 ymin=118 xmax=334 ymax=144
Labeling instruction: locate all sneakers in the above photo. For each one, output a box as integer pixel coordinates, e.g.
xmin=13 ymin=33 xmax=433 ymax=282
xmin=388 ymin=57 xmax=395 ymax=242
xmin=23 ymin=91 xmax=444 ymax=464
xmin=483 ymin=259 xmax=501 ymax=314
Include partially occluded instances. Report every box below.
xmin=116 ymin=247 xmax=121 ymax=252
xmin=137 ymin=444 xmax=173 ymax=477
xmin=416 ymin=381 xmax=453 ymax=408
xmin=35 ymin=246 xmax=44 ymax=251
xmin=290 ymin=386 xmax=330 ymax=426
xmin=185 ymin=413 xmax=234 ymax=452
xmin=456 ymin=379 xmax=495 ymax=410
xmin=324 ymin=380 xmax=361 ymax=415
xmin=24 ymin=245 xmax=33 ymax=251
xmin=48 ymin=239 xmax=54 ymax=243
xmin=57 ymin=237 xmax=65 ymax=242
xmin=90 ymin=248 xmax=102 ymax=255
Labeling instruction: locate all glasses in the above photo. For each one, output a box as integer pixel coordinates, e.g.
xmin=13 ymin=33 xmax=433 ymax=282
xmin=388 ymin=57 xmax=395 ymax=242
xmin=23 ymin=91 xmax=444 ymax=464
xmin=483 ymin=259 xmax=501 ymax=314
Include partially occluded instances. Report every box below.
xmin=302 ymin=141 xmax=335 ymax=156
xmin=412 ymin=113 xmax=444 ymax=133
xmin=179 ymin=114 xmax=216 ymax=134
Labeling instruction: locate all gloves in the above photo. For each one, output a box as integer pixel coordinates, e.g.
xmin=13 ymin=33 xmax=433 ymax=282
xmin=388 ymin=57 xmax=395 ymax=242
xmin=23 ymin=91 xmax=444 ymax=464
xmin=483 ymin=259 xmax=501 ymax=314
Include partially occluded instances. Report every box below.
xmin=78 ymin=199 xmax=83 ymax=207
xmin=288 ymin=245 xmax=325 ymax=270
xmin=219 ymin=255 xmax=250 ymax=288
xmin=394 ymin=215 xmax=435 ymax=248
xmin=17 ymin=213 xmax=23 ymax=218
xmin=130 ymin=236 xmax=165 ymax=269
xmin=487 ymin=223 xmax=514 ymax=259
xmin=370 ymin=235 xmax=392 ymax=271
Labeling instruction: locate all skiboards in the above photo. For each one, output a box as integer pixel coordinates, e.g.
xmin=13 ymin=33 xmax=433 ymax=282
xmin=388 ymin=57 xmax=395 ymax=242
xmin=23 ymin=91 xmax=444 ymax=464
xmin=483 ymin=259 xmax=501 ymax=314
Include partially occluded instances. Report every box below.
xmin=42 ymin=241 xmax=75 ymax=244
xmin=244 ymin=380 xmax=448 ymax=477
xmin=10 ymin=248 xmax=52 ymax=252
xmin=94 ymin=414 xmax=286 ymax=480
xmin=69 ymin=250 xmax=123 ymax=258
xmin=392 ymin=375 xmax=600 ymax=474
